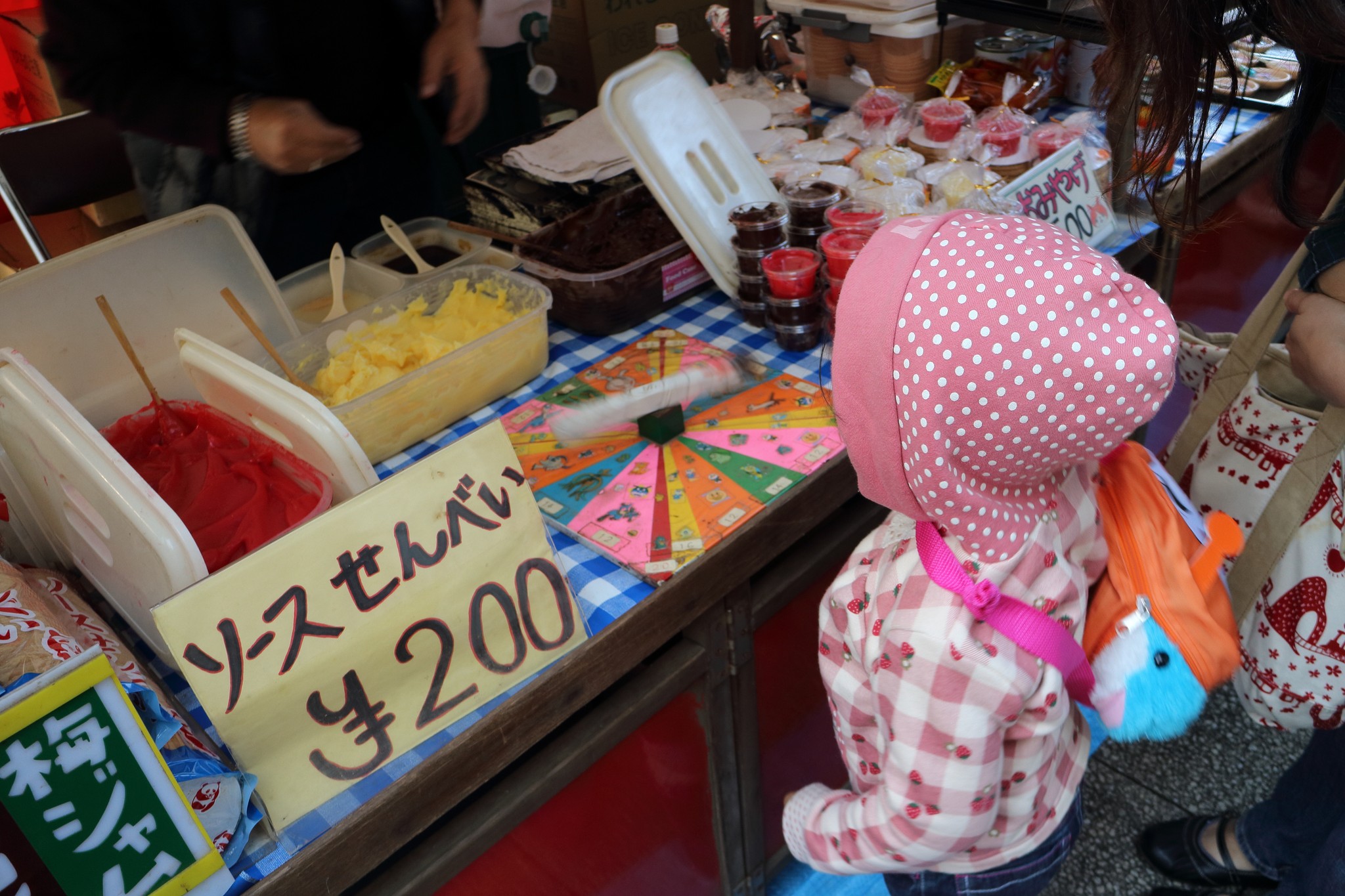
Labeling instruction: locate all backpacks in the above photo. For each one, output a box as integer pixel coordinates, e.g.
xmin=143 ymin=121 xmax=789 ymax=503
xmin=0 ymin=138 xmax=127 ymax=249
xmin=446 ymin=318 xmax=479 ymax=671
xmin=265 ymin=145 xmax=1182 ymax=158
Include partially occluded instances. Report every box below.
xmin=920 ymin=433 xmax=1246 ymax=746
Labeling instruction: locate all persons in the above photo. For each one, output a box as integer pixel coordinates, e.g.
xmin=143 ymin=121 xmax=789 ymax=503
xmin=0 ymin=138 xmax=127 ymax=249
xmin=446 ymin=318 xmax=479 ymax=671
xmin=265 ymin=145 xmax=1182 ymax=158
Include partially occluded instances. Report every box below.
xmin=103 ymin=1 xmax=509 ymax=296
xmin=1126 ymin=188 xmax=1345 ymax=895
xmin=787 ymin=205 xmax=1184 ymax=895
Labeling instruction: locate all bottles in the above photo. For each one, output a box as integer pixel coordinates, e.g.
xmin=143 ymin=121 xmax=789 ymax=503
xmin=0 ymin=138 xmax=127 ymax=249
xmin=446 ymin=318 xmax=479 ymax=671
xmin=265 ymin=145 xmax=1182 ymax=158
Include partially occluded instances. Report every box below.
xmin=653 ymin=22 xmax=692 ymax=65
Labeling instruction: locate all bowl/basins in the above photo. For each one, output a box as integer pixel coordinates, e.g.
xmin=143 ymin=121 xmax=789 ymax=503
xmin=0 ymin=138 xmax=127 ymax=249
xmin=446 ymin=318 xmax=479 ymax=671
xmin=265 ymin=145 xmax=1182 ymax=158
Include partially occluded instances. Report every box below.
xmin=710 ymin=76 xmax=1098 ymax=358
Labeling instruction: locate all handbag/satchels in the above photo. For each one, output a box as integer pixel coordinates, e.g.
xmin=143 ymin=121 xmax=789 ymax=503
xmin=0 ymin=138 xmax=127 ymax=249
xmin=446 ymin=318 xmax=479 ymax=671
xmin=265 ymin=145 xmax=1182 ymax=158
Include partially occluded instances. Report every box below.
xmin=1152 ymin=315 xmax=1345 ymax=734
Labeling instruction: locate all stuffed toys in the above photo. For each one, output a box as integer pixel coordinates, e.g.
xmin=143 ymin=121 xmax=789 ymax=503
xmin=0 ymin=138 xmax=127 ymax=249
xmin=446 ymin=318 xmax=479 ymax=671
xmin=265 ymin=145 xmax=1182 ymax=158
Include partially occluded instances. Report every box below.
xmin=1076 ymin=611 xmax=1210 ymax=754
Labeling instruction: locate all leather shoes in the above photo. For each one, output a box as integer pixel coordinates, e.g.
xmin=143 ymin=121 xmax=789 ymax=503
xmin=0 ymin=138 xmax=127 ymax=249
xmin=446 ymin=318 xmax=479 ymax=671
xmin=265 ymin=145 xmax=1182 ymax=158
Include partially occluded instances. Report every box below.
xmin=1137 ymin=806 xmax=1279 ymax=896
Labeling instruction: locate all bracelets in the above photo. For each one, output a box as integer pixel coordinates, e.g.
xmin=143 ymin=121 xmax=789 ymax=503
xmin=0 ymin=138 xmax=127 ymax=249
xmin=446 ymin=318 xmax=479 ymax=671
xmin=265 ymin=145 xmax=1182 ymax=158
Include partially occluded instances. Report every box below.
xmin=229 ymin=98 xmax=255 ymax=163
